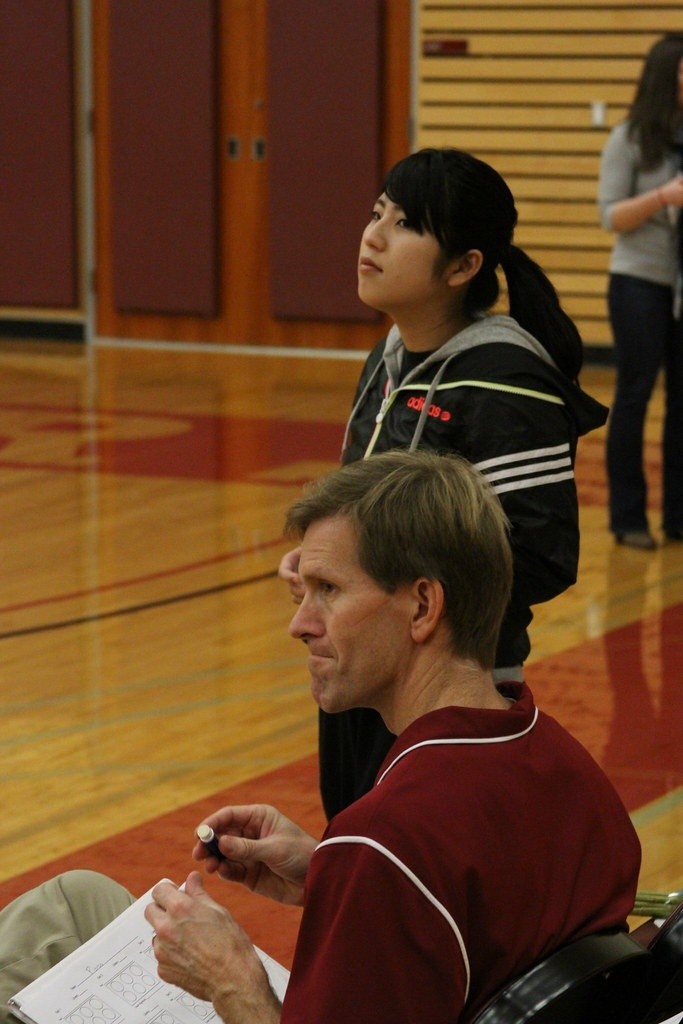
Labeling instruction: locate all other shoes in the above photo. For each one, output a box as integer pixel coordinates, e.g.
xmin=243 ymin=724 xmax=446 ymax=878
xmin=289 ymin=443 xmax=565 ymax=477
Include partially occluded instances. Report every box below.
xmin=617 ymin=530 xmax=656 ymax=549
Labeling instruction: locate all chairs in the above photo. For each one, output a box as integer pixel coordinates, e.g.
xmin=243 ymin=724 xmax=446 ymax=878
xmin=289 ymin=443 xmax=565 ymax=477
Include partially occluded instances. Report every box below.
xmin=476 ymin=896 xmax=683 ymax=1024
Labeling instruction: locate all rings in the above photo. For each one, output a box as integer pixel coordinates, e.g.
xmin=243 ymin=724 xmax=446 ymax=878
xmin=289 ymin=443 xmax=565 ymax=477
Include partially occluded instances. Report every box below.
xmin=151 ymin=933 xmax=158 ymax=945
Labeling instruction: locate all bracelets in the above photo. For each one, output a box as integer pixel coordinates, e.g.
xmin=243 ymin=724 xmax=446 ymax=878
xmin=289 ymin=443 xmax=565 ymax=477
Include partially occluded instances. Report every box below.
xmin=656 ymin=189 xmax=668 ymax=209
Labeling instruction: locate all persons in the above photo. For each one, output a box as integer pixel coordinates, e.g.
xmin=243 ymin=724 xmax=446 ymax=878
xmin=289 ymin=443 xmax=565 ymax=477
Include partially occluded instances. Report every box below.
xmin=0 ymin=449 xmax=642 ymax=1024
xmin=598 ymin=34 xmax=683 ymax=547
xmin=280 ymin=146 xmax=587 ymax=819
